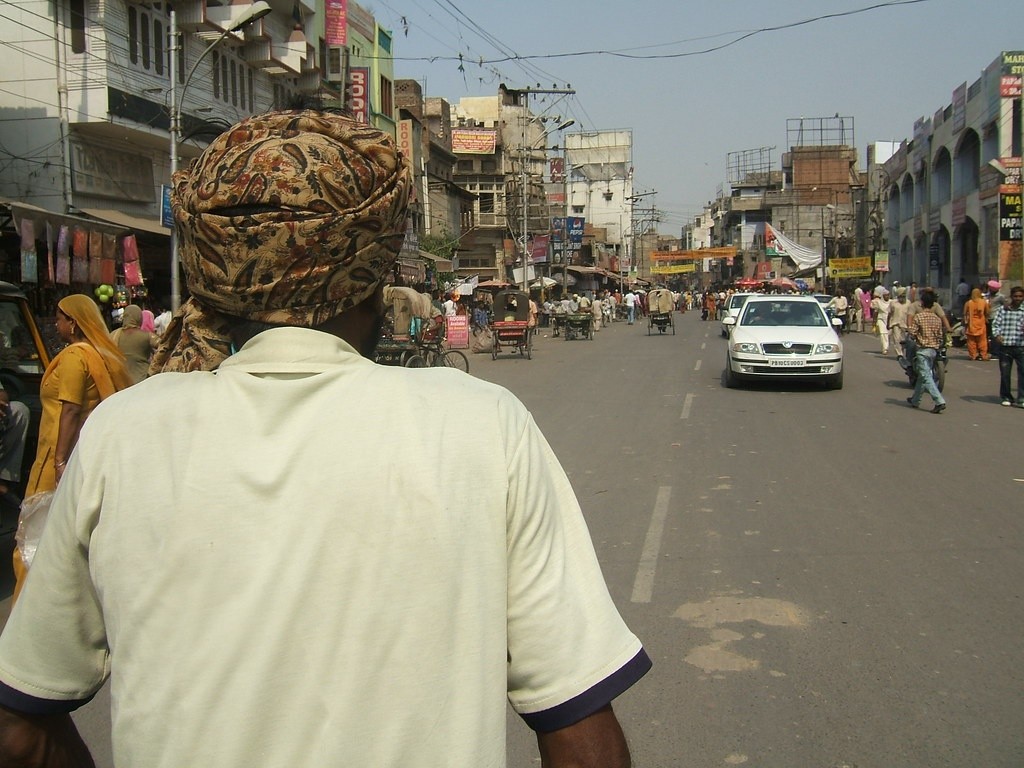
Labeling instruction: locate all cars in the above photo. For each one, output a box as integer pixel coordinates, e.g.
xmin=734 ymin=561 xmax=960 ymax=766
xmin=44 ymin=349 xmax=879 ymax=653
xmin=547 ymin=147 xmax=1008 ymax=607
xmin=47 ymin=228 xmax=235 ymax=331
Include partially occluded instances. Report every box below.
xmin=810 ymin=294 xmax=833 ymax=309
xmin=720 ymin=292 xmax=763 ymax=340
xmin=721 ymin=295 xmax=844 ymax=390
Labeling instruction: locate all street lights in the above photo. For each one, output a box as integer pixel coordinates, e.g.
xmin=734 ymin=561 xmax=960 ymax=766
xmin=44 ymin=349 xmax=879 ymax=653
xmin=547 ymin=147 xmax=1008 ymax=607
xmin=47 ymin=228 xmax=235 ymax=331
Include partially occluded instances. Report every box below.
xmin=826 ymin=203 xmax=859 ymax=286
xmin=619 ymin=214 xmax=647 ymax=298
xmin=165 ymin=1 xmax=273 ymax=318
xmin=520 ymin=119 xmax=578 ymax=291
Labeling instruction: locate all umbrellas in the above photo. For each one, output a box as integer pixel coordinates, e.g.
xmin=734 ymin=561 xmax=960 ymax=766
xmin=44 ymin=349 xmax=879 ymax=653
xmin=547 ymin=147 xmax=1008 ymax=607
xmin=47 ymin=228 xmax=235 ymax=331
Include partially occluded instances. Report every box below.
xmin=478 ymin=280 xmax=511 ymax=289
xmin=768 ymin=277 xmax=792 ymax=291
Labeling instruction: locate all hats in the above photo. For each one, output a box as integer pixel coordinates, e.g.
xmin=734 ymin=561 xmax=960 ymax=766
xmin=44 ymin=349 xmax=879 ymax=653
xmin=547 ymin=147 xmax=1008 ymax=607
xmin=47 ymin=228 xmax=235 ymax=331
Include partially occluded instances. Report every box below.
xmin=452 ymin=293 xmax=461 ymax=302
xmin=170 ymin=109 xmax=410 ymax=325
xmin=987 ymin=279 xmax=1002 ymax=292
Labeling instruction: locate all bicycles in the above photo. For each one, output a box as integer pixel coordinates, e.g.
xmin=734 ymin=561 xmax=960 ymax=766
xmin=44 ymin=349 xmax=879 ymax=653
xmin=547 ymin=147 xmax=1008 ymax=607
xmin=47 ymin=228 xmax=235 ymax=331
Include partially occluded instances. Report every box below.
xmin=403 ymin=334 xmax=471 ymax=374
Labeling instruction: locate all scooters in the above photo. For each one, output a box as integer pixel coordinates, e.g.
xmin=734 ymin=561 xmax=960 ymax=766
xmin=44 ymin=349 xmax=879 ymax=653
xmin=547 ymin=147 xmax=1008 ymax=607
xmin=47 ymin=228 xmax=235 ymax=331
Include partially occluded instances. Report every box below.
xmin=895 ymin=329 xmax=953 ymax=393
xmin=949 ymin=317 xmax=969 ymax=348
xmin=823 ymin=304 xmax=841 ymax=334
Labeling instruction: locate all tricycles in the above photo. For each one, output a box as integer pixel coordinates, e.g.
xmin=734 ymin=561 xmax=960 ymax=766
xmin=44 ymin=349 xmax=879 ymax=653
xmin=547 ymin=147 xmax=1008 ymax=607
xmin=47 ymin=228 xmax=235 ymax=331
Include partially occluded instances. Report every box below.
xmin=647 ymin=289 xmax=676 ymax=335
xmin=487 ymin=290 xmax=534 ymax=360
xmin=550 ymin=307 xmax=570 ymax=338
xmin=373 ymin=284 xmax=429 ymax=367
xmin=564 ymin=308 xmax=594 ymax=340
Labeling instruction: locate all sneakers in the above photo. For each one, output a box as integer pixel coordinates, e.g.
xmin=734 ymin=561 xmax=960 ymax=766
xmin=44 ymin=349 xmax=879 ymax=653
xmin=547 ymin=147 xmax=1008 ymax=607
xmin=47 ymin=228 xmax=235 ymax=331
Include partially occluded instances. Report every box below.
xmin=930 ymin=404 xmax=946 ymax=413
xmin=906 ymin=397 xmax=920 ymax=407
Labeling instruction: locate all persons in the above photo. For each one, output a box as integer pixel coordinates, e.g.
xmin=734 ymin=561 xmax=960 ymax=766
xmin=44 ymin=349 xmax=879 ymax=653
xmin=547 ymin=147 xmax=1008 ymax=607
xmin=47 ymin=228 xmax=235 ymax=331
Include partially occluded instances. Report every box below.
xmin=986 ymin=280 xmax=1008 ymax=358
xmin=672 ymin=286 xmax=810 ymax=321
xmin=0 ymin=110 xmax=653 ymax=768
xmin=964 ymin=288 xmax=991 ymax=361
xmin=907 ymin=292 xmax=946 ymax=413
xmin=13 ymin=294 xmax=134 ymax=610
xmin=827 ymin=281 xmax=912 ymax=361
xmin=907 ymin=287 xmax=953 ymax=334
xmin=110 ymin=305 xmax=163 ymax=385
xmin=956 ymin=277 xmax=968 ymax=314
xmin=991 ymin=287 xmax=1024 ymax=408
xmin=421 ymin=289 xmax=648 ymax=353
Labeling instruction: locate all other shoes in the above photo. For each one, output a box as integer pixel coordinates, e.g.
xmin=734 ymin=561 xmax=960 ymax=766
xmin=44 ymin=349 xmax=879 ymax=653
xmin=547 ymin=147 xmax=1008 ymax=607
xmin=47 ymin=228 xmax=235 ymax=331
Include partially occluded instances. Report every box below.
xmin=882 ymin=350 xmax=888 ymax=354
xmin=1001 ymin=398 xmax=1024 ymax=408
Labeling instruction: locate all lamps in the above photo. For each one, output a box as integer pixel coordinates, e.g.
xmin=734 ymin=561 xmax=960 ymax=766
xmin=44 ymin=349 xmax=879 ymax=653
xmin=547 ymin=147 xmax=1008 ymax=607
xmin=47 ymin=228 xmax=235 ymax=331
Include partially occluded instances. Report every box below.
xmin=143 ymin=88 xmax=163 ymax=95
xmin=194 ymin=105 xmax=214 ymax=113
